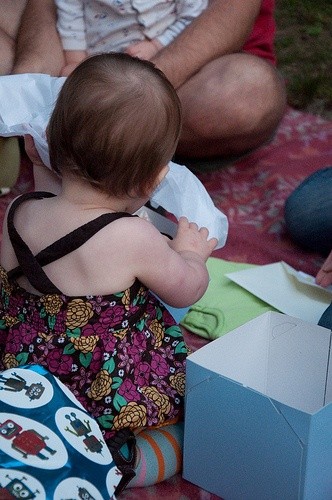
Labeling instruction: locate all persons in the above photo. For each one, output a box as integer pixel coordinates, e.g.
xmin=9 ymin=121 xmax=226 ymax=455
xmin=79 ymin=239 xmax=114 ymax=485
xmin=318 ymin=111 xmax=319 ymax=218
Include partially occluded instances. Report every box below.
xmin=2 ymin=0 xmax=288 ymax=181
xmin=279 ymin=162 xmax=332 ymax=293
xmin=0 ymin=50 xmax=220 ymax=488
xmin=45 ymin=0 xmax=208 ymax=77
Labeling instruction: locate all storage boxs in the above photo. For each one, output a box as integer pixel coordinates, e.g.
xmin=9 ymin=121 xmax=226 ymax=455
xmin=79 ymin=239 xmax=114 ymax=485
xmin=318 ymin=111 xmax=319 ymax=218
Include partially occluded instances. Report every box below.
xmin=181 ymin=310 xmax=332 ymax=500
